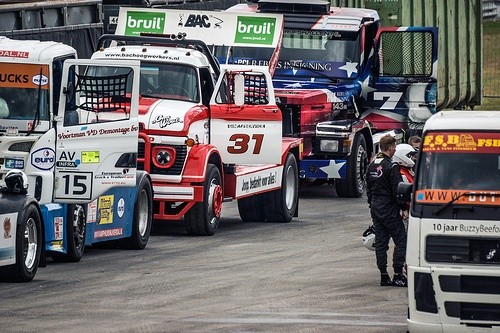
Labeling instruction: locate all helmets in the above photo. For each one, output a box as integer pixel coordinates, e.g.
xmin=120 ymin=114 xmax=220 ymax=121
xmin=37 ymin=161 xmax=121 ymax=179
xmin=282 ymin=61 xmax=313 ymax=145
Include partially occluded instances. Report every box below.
xmin=392 ymin=143 xmax=418 ymax=168
xmin=362 ymin=224 xmax=389 ymax=251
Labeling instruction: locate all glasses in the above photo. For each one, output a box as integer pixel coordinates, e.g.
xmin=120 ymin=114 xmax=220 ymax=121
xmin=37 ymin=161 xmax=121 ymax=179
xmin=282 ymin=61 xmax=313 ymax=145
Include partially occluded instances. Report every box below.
xmin=413 ymin=146 xmax=420 ymax=151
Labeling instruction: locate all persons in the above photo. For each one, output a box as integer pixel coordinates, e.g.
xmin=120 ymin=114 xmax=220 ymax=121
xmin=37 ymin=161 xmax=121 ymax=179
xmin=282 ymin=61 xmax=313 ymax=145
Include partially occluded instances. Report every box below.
xmin=392 ymin=137 xmax=420 ymax=220
xmin=366 ymin=135 xmax=407 ymax=286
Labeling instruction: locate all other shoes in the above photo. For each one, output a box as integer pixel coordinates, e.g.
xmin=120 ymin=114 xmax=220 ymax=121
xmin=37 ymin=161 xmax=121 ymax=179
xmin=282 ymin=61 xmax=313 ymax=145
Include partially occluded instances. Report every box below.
xmin=380 ymin=274 xmax=395 ymax=287
xmin=394 ymin=274 xmax=408 ymax=286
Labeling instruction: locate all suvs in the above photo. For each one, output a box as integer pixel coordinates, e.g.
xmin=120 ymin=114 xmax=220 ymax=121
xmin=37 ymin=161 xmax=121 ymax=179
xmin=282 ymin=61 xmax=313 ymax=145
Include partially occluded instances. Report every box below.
xmin=211 ymin=0 xmax=440 ymax=199
xmin=0 ymin=35 xmax=155 ymax=283
xmin=79 ymin=7 xmax=304 ymax=237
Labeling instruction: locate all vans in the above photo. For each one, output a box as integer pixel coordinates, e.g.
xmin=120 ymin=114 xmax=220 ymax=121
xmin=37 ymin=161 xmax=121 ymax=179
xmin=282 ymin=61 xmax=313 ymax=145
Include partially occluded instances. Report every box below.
xmin=396 ymin=109 xmax=499 ymax=333
